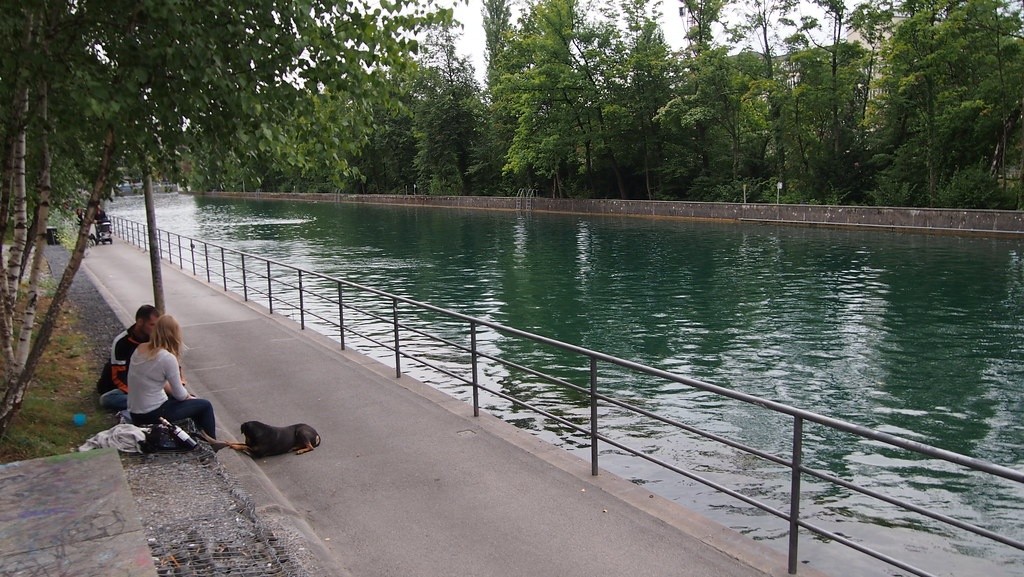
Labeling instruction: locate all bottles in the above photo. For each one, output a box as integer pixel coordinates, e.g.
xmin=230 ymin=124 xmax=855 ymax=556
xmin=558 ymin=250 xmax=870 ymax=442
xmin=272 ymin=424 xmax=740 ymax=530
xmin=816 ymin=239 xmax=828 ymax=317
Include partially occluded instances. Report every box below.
xmin=159 ymin=417 xmax=197 ymax=450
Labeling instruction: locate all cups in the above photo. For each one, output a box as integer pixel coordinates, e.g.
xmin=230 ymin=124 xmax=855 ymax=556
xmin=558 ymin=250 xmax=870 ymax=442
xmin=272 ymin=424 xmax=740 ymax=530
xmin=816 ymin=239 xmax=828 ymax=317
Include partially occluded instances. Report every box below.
xmin=73 ymin=414 xmax=86 ymax=427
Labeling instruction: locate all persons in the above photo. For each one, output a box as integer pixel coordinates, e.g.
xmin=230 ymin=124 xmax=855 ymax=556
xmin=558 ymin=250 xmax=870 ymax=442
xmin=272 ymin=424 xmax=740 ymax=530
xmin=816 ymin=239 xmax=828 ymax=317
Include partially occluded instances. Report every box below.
xmin=127 ymin=314 xmax=216 ymax=441
xmin=94 ymin=204 xmax=106 ymax=225
xmin=100 ymin=305 xmax=185 ymax=412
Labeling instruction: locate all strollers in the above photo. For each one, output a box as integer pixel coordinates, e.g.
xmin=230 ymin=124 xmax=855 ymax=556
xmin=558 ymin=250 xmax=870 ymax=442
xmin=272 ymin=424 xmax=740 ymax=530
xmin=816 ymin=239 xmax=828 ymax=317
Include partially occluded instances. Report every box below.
xmin=93 ymin=220 xmax=113 ymax=246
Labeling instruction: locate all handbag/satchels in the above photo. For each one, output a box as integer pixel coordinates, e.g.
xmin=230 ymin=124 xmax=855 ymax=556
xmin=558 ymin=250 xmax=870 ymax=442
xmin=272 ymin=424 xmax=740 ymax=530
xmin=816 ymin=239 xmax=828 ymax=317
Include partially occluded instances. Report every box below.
xmin=139 ymin=418 xmax=197 ymax=450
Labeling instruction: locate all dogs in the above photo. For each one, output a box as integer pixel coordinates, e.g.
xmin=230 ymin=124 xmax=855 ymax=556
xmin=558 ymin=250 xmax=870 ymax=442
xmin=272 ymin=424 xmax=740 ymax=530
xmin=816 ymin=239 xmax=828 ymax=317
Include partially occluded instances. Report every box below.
xmin=225 ymin=421 xmax=321 ymax=458
